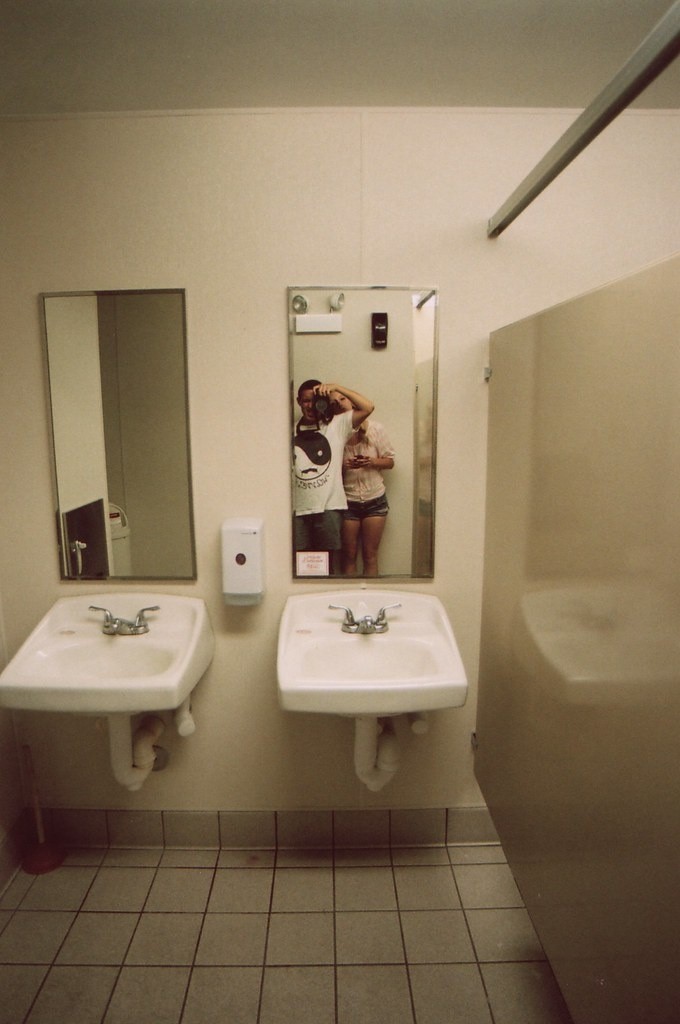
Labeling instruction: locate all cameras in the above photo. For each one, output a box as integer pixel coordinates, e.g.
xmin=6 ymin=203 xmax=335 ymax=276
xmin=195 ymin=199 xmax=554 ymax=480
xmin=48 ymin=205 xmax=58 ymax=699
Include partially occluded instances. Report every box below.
xmin=313 ymin=389 xmax=330 ymax=418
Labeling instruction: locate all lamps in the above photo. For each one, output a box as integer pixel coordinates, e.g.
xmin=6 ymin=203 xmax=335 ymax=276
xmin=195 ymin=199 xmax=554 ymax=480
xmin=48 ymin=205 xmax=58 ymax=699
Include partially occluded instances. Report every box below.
xmin=292 ymin=295 xmax=310 ymax=314
xmin=328 ymin=293 xmax=345 ymax=312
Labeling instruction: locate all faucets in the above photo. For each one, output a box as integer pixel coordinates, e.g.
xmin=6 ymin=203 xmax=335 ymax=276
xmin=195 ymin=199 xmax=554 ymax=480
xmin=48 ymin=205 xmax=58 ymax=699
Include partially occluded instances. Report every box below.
xmin=357 ymin=615 xmax=376 ymax=633
xmin=102 ymin=618 xmax=122 ymax=635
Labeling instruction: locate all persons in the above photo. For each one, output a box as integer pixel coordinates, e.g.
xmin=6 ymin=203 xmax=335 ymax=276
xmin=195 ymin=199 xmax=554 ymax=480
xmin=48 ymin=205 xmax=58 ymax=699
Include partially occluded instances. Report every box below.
xmin=328 ymin=390 xmax=395 ymax=577
xmin=292 ymin=381 xmax=374 ymax=576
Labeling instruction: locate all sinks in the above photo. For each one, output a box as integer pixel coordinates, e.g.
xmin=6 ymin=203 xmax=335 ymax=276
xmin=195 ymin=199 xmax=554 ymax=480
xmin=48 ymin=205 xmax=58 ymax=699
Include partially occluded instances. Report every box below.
xmin=277 ymin=590 xmax=468 ymax=713
xmin=0 ymin=593 xmax=215 ymax=711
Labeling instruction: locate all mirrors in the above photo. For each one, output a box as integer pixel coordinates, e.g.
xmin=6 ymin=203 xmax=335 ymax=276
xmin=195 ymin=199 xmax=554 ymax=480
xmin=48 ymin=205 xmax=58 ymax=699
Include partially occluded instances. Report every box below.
xmin=36 ymin=287 xmax=198 ymax=583
xmin=286 ymin=285 xmax=437 ymax=578
